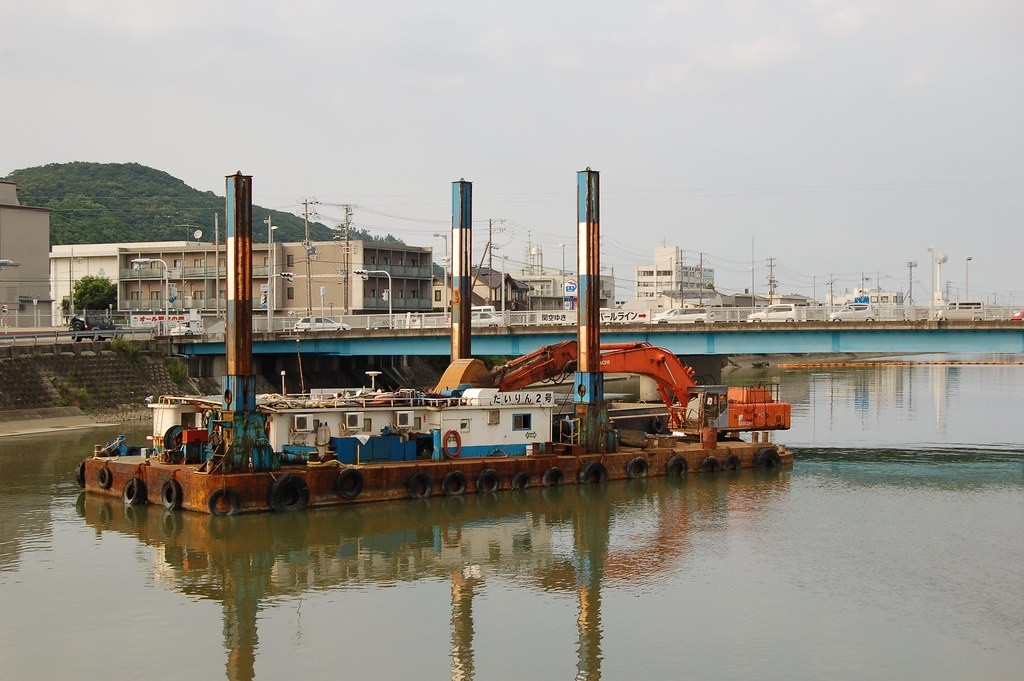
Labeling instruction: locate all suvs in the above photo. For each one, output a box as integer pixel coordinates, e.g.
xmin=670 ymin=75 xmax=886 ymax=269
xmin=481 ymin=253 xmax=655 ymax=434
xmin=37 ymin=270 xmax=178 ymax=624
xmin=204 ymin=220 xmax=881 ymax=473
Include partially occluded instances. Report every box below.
xmin=69 ymin=314 xmax=123 ymax=342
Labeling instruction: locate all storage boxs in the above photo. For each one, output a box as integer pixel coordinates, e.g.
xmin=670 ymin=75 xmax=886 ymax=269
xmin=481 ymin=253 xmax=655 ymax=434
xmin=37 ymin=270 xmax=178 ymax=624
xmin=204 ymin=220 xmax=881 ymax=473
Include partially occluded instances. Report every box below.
xmin=183 ymin=430 xmax=207 ymax=443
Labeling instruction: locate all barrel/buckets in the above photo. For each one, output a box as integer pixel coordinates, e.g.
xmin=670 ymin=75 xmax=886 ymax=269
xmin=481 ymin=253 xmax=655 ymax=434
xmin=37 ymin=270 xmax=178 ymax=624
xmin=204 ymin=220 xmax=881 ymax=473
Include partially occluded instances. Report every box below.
xmin=526 ymin=445 xmax=532 ymax=456
xmin=702 ymin=427 xmax=717 ymax=449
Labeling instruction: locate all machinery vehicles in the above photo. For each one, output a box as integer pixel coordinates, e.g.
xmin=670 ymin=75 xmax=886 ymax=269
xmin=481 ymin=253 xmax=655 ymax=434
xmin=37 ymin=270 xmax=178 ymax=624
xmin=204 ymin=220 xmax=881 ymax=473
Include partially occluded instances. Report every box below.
xmin=433 ymin=339 xmax=791 ymax=442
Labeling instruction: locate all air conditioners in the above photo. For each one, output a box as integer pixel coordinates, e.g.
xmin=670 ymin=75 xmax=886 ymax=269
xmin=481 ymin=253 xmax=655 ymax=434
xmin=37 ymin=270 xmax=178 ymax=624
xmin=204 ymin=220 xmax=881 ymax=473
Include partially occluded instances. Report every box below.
xmin=394 ymin=410 xmax=415 ymax=428
xmin=292 ymin=414 xmax=314 ymax=431
xmin=344 ymin=411 xmax=365 ymax=429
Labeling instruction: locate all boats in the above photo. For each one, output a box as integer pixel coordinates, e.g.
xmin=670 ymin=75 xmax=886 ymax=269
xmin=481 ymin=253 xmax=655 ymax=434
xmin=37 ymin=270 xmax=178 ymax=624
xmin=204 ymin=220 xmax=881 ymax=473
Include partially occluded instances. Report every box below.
xmin=78 ymin=173 xmax=795 ymax=515
xmin=752 ymin=361 xmax=769 ymax=367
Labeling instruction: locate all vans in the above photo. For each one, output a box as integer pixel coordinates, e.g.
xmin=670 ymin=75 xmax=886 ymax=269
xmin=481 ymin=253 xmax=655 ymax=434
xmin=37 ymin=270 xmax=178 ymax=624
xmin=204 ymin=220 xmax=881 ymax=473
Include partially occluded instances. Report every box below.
xmin=934 ymin=302 xmax=985 ymax=321
xmin=471 ymin=306 xmax=495 ymax=315
xmin=650 ymin=308 xmax=707 ymax=324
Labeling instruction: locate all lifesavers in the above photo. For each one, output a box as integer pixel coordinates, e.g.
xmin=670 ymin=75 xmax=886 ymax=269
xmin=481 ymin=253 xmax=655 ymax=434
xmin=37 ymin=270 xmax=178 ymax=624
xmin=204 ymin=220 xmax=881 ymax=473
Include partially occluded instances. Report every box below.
xmin=578 ymin=483 xmax=607 ymax=503
xmin=336 ymin=511 xmax=362 ymax=538
xmin=268 ymin=473 xmax=310 ymax=512
xmin=76 ymin=491 xmax=86 ymax=517
xmin=336 ymin=469 xmax=363 ymax=499
xmin=511 ymin=490 xmax=532 ymax=507
xmin=441 ymin=495 xmax=466 ymax=518
xmin=476 ymin=492 xmax=499 ymax=511
xmin=406 ymin=466 xmax=564 ymax=498
xmin=208 ymin=515 xmax=241 ymax=540
xmin=543 ymin=487 xmax=563 ymax=502
xmin=578 ymin=448 xmax=783 ymax=485
xmin=207 ymin=488 xmax=240 ymax=516
xmin=269 ymin=510 xmax=308 ymax=548
xmin=123 ymin=504 xmax=147 ymax=524
xmin=97 ymin=467 xmax=183 ymax=511
xmin=160 ymin=510 xmax=183 ymax=539
xmin=75 ymin=461 xmax=85 ymax=488
xmin=409 ymin=499 xmax=431 ymax=522
xmin=96 ymin=503 xmax=113 ymax=526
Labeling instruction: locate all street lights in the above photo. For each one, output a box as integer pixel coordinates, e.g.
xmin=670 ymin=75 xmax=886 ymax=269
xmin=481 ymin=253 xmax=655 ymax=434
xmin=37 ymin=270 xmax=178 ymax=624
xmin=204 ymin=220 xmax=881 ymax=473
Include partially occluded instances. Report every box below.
xmin=501 ymin=255 xmax=508 ymax=321
xmin=559 ymin=244 xmax=566 ymax=309
xmin=433 ymin=234 xmax=448 ymax=313
xmin=812 ymin=275 xmax=815 ymax=304
xmin=264 ymin=220 xmax=278 ymax=331
xmin=965 ymin=256 xmax=971 ymax=302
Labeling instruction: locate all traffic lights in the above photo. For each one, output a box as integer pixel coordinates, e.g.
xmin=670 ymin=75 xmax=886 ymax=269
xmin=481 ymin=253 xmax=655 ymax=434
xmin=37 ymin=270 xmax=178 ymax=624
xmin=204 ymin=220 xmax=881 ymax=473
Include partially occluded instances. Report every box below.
xmin=2 ymin=305 xmax=8 ymax=315
xmin=354 ymin=270 xmax=369 ymax=281
xmin=280 ymin=272 xmax=293 ymax=283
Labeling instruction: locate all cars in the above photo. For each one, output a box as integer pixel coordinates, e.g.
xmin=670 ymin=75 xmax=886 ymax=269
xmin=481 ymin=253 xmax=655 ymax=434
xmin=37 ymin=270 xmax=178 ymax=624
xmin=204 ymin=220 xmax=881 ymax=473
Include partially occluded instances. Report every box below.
xmin=828 ymin=303 xmax=876 ymax=322
xmin=170 ymin=322 xmax=204 ymax=335
xmin=448 ymin=313 xmax=503 ymax=327
xmin=653 ymin=309 xmax=677 ymax=317
xmin=1010 ymin=307 xmax=1024 ymax=321
xmin=295 ymin=317 xmax=351 ymax=331
xmin=747 ymin=304 xmax=799 ymax=322
